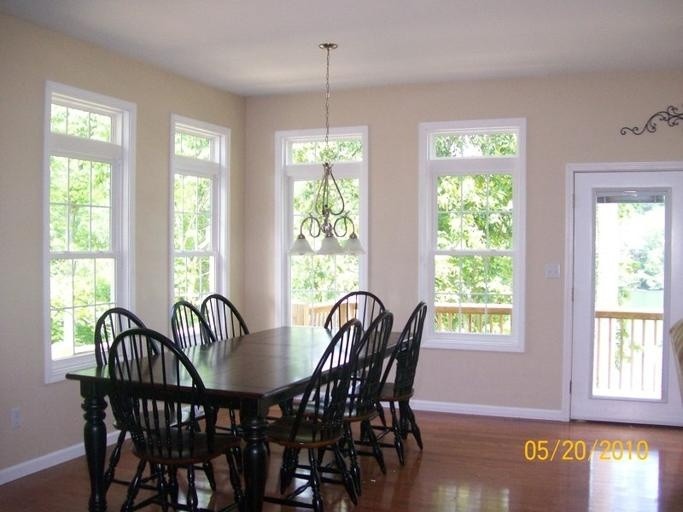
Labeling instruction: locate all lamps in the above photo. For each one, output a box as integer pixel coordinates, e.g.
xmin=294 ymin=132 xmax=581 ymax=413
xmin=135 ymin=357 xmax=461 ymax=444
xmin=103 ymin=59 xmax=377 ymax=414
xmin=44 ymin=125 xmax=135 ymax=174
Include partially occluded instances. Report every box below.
xmin=288 ymin=43 xmax=367 ymax=257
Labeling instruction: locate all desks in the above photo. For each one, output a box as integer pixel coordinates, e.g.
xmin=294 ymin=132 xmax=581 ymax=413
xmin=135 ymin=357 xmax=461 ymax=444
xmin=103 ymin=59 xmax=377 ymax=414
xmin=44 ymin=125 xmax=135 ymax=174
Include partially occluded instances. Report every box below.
xmin=65 ymin=326 xmax=413 ymax=512
